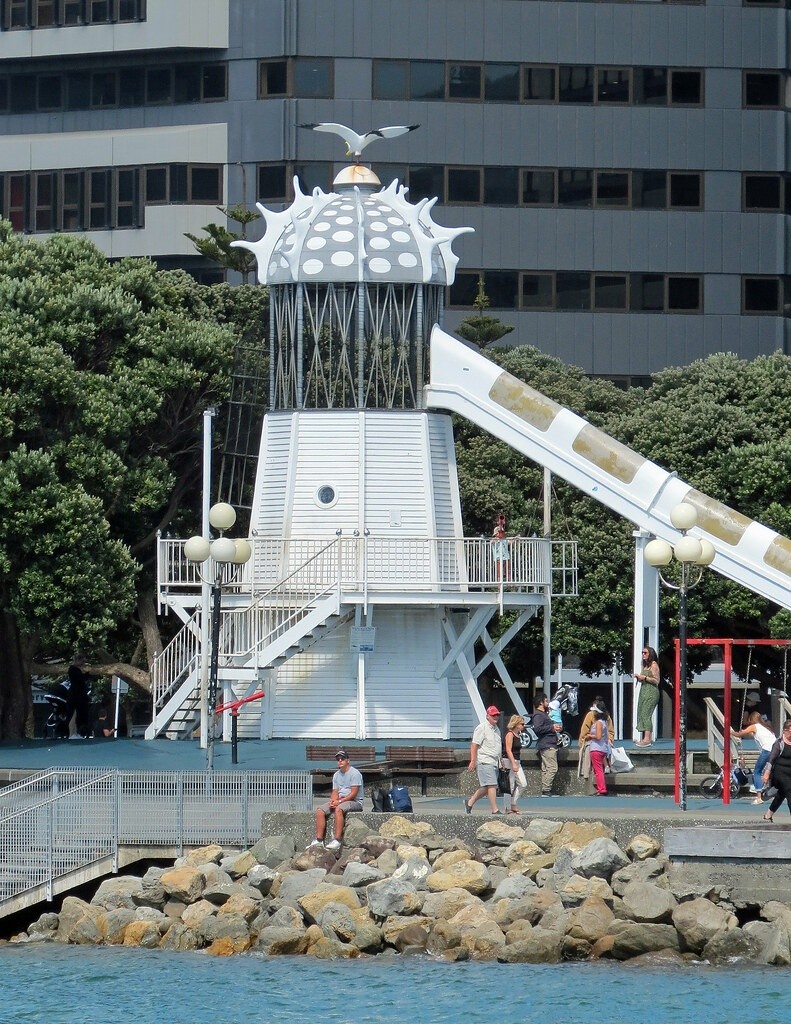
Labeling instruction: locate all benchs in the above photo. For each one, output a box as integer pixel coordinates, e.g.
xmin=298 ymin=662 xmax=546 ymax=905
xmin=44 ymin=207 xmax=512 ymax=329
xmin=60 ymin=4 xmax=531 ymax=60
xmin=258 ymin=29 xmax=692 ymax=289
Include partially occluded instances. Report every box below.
xmin=305 ymin=745 xmax=385 ymax=776
xmin=383 ymin=745 xmax=461 ymax=795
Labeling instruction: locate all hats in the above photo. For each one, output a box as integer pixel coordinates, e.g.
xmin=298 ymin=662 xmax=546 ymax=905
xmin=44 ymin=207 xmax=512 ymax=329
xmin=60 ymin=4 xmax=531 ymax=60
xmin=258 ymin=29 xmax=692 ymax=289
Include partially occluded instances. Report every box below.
xmin=487 ymin=705 xmax=501 ymax=715
xmin=335 ymin=751 xmax=348 ymax=758
xmin=747 ymin=692 xmax=760 ymax=701
xmin=547 ymin=700 xmax=560 ymax=710
xmin=589 ymin=705 xmax=603 ymax=713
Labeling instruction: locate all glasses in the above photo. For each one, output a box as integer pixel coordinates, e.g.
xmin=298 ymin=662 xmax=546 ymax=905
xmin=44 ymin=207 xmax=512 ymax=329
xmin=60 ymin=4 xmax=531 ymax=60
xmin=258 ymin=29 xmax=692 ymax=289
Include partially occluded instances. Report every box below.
xmin=337 ymin=756 xmax=347 ymax=761
xmin=520 ymin=723 xmax=524 ymax=726
xmin=642 ymin=651 xmax=649 ymax=655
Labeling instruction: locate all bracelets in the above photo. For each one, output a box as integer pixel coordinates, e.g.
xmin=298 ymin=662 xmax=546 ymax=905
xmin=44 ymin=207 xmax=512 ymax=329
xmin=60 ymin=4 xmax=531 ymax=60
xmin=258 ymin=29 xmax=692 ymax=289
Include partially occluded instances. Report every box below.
xmin=339 ymin=800 xmax=341 ymax=803
xmin=645 ymin=676 xmax=647 ymax=681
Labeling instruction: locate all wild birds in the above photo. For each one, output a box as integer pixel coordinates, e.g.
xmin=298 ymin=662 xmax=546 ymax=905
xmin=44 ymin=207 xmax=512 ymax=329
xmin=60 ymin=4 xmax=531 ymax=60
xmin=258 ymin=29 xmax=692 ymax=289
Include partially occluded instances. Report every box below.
xmin=294 ymin=123 xmax=422 ymax=161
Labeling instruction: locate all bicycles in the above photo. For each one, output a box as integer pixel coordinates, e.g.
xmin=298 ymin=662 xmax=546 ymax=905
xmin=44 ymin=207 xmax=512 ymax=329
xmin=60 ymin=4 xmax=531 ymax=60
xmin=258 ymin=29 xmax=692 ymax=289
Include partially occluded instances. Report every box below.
xmin=699 ymin=757 xmax=749 ymax=802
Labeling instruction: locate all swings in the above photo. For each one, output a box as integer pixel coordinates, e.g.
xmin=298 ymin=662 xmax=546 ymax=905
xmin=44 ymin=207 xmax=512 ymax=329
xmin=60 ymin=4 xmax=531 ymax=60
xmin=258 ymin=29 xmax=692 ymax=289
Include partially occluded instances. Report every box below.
xmin=733 ymin=645 xmax=788 ymax=785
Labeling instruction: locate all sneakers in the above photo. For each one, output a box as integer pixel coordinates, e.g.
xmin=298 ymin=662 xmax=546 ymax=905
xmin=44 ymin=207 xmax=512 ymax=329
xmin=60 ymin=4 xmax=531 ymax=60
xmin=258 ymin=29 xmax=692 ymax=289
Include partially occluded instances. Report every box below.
xmin=306 ymin=840 xmax=325 ymax=849
xmin=325 ymin=839 xmax=342 ymax=849
xmin=69 ymin=733 xmax=83 ymax=739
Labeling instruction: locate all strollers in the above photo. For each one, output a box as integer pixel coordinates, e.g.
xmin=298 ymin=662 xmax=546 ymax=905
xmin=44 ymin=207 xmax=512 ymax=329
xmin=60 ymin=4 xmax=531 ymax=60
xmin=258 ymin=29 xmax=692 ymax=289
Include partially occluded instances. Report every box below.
xmin=517 ymin=680 xmax=580 ymax=749
xmin=41 ymin=664 xmax=91 ymax=739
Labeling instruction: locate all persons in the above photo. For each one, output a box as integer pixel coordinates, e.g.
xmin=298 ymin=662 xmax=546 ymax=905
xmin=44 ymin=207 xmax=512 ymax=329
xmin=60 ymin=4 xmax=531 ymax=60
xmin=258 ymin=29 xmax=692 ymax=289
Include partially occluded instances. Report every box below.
xmin=306 ymin=751 xmax=364 ymax=849
xmin=548 ymin=701 xmax=563 ymax=746
xmin=762 ymin=719 xmax=791 ymax=821
xmin=493 ymin=516 xmax=520 ymax=593
xmin=733 ymin=711 xmax=776 ymax=805
xmin=67 ymin=655 xmax=96 ymax=739
xmin=463 ymin=706 xmax=505 ymax=814
xmin=93 ymin=709 xmax=114 ymax=737
xmin=633 ymin=646 xmax=660 ymax=747
xmin=532 ymin=693 xmax=562 ymax=798
xmin=503 ymin=715 xmax=527 ymax=814
xmin=578 ymin=696 xmax=615 ymax=795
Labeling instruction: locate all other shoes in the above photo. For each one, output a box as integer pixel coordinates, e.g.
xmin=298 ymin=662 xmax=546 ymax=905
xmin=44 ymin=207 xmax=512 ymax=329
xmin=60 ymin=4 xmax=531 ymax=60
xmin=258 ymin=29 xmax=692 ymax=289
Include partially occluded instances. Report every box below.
xmin=492 ymin=810 xmax=502 ymax=814
xmin=763 ymin=815 xmax=773 ymax=822
xmin=555 ymin=740 xmax=563 ymax=747
xmin=589 ymin=791 xmax=608 ymax=797
xmin=635 ymin=741 xmax=652 ymax=747
xmin=751 ymin=798 xmax=763 ymax=805
xmin=541 ymin=791 xmax=559 ymax=798
xmin=462 ymin=799 xmax=471 ymax=815
xmin=505 ymin=810 xmax=523 ymax=815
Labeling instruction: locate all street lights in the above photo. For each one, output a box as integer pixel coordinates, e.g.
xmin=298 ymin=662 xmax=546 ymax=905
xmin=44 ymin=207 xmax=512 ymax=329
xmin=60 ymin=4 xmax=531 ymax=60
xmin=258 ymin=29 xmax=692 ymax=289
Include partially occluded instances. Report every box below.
xmin=183 ymin=503 xmax=252 ymax=800
xmin=643 ymin=502 xmax=717 ymax=810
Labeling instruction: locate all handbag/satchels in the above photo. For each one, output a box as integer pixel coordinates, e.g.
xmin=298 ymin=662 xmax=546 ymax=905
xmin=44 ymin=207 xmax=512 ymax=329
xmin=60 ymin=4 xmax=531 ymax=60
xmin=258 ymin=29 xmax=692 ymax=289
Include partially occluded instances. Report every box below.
xmin=385 ymin=783 xmax=412 ymax=813
xmin=609 ymin=743 xmax=634 ymax=773
xmin=370 ymin=787 xmax=387 ymax=812
